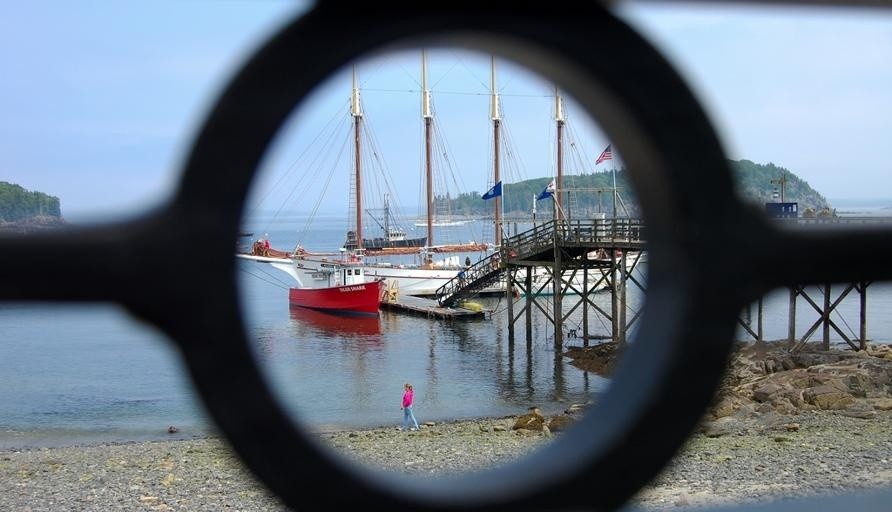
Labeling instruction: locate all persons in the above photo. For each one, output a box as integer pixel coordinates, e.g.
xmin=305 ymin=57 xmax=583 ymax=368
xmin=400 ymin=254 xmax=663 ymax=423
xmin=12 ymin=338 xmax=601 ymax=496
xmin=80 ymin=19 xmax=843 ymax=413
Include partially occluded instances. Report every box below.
xmin=490 ymin=253 xmax=501 ymax=270
xmin=263 ymin=239 xmax=270 ymax=257
xmin=400 ymin=383 xmax=420 ymax=431
xmin=465 ymin=257 xmax=471 ymax=267
xmin=457 ymin=268 xmax=466 ymax=288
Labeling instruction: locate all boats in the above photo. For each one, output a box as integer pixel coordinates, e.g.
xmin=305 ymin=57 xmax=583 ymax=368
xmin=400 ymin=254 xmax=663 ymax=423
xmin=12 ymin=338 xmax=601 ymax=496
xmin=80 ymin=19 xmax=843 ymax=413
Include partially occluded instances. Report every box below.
xmin=235 ymin=231 xmax=255 ymax=236
xmin=282 ymin=262 xmax=386 ymax=315
xmin=288 ymin=304 xmax=383 ymax=339
xmin=338 ymin=194 xmax=427 ymax=248
xmin=234 ymin=241 xmax=251 ymax=250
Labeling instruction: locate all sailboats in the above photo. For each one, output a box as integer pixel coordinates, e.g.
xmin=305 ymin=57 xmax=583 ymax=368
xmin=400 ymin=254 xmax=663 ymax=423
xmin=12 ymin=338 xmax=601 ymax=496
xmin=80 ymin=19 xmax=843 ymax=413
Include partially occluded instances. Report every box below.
xmin=195 ymin=45 xmax=648 ymax=299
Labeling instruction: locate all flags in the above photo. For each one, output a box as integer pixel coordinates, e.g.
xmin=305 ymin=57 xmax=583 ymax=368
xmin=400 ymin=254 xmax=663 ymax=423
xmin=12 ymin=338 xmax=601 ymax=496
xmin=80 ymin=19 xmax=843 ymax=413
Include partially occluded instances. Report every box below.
xmin=534 ymin=177 xmax=556 ymax=201
xmin=595 ymin=143 xmax=612 ymax=165
xmin=481 ymin=181 xmax=501 ymax=200
